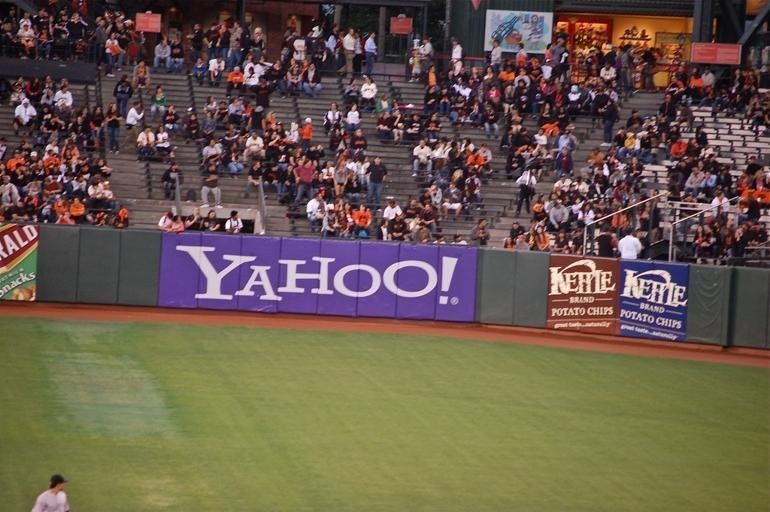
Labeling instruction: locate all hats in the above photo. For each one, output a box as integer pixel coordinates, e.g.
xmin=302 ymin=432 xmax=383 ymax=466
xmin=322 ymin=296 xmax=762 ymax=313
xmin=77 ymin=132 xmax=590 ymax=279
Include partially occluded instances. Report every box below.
xmin=51 ymin=475 xmax=67 ymax=484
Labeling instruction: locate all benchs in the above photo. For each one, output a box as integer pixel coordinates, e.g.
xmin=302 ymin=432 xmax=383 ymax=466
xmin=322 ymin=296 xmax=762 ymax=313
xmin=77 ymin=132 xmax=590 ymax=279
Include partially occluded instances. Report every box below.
xmin=0 ymin=64 xmax=770 ymax=267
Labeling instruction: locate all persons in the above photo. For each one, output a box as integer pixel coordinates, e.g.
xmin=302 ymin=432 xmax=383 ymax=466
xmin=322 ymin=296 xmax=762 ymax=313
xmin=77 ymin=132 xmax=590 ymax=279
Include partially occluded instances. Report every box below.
xmin=0 ymin=1 xmax=769 ymax=268
xmin=30 ymin=473 xmax=71 ymax=512
